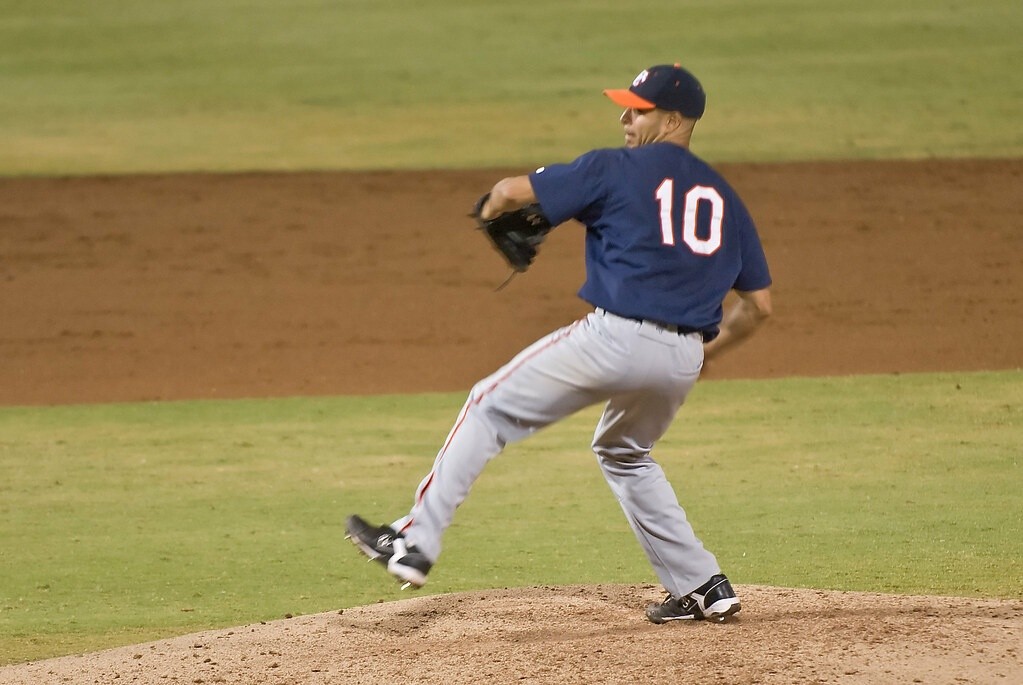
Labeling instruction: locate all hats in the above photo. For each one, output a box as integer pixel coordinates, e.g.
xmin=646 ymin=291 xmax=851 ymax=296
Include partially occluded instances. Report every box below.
xmin=599 ymin=61 xmax=707 ymax=121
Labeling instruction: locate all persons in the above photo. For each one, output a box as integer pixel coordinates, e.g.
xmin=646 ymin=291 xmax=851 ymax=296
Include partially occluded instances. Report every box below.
xmin=344 ymin=62 xmax=774 ymax=626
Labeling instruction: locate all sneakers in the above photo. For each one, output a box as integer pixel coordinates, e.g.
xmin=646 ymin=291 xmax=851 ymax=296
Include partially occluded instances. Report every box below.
xmin=345 ymin=512 xmax=431 ymax=591
xmin=644 ymin=574 xmax=741 ymax=624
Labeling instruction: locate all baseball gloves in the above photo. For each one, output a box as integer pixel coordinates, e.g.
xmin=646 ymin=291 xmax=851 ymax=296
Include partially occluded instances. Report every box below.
xmin=470 ymin=192 xmax=552 ymax=274
